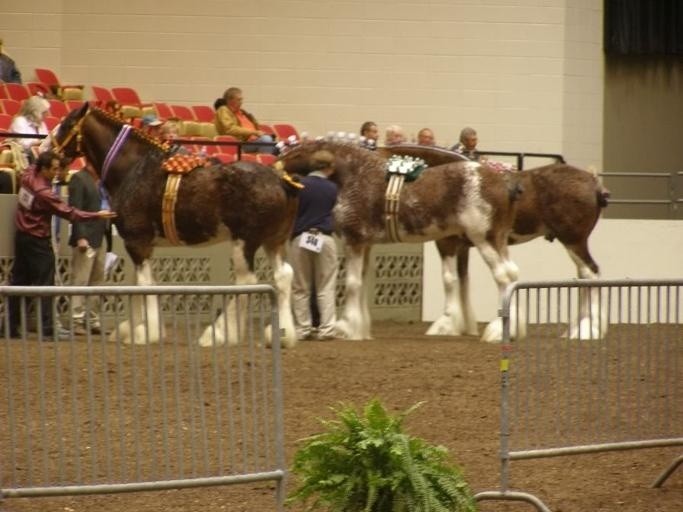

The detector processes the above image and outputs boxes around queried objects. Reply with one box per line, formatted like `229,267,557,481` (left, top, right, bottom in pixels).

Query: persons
7,95,51,152
0,53,22,83
0,152,119,340
286,149,339,340
449,127,480,162
215,86,275,155
384,125,404,146
418,128,434,147
67,156,114,335
139,115,168,145
360,121,379,142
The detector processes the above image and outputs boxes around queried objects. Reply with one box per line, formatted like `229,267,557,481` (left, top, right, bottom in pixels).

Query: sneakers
43,326,112,336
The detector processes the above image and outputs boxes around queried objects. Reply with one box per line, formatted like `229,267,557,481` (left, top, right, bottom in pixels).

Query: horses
37,101,302,351
371,142,611,340
267,138,522,344
